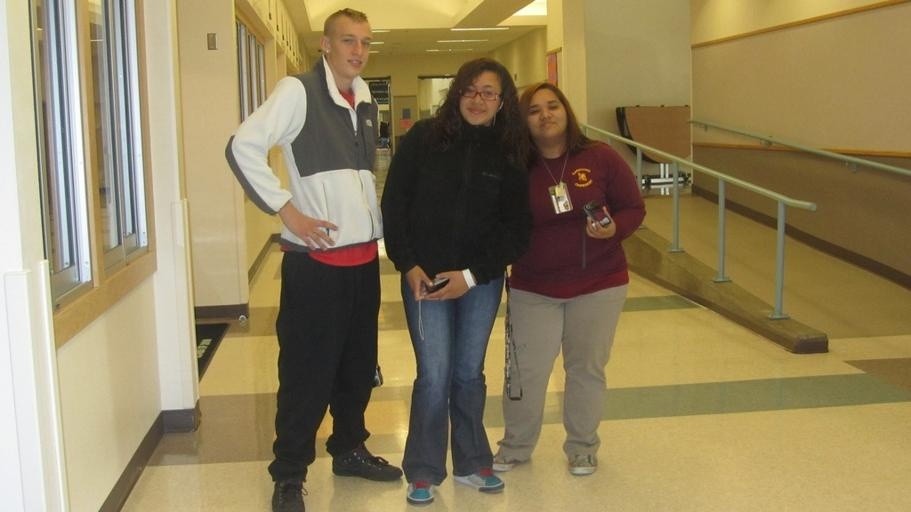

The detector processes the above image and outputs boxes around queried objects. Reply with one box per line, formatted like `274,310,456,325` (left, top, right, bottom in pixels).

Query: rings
311,232,315,238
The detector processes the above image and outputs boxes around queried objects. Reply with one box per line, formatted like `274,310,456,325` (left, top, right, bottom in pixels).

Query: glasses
458,86,503,100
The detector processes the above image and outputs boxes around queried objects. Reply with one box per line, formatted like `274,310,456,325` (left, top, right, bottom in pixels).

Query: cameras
427,278,448,293
582,200,611,227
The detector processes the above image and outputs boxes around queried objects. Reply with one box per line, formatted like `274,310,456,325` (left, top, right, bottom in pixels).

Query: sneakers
270,478,309,512
406,478,437,504
454,467,505,494
331,446,404,483
568,454,598,476
489,453,533,471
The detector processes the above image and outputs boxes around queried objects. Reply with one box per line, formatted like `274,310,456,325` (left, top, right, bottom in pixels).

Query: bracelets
462,269,475,289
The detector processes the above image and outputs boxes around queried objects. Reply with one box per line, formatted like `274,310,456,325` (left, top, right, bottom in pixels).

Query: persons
490,82,645,474
224,7,404,512
381,57,535,506
